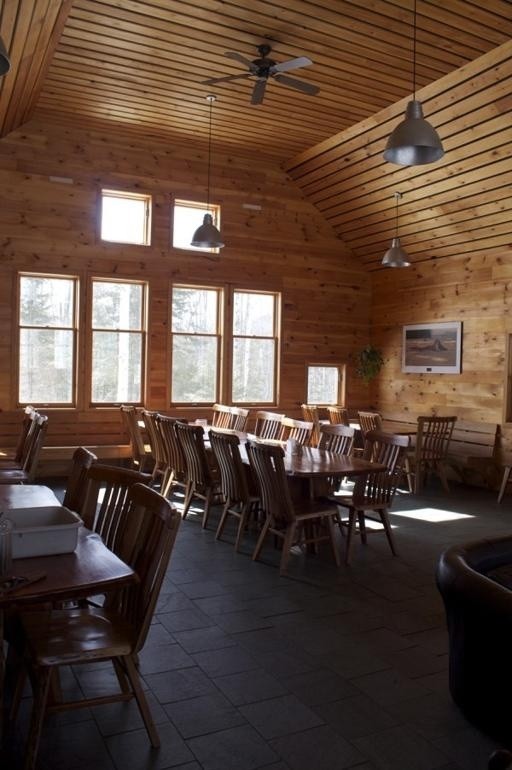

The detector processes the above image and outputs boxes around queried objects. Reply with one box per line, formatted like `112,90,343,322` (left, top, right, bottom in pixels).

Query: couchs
430,533,512,749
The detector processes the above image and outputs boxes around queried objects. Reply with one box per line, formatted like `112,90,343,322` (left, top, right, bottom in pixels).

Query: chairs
358,409,388,460
244,438,343,578
210,404,234,430
300,404,325,447
4,480,181,763
280,418,313,445
0,405,39,458
398,416,456,497
318,424,356,455
208,430,261,552
151,411,189,498
491,421,511,504
141,412,165,496
0,415,48,482
0,484,142,605
171,419,220,529
62,448,95,515
255,410,284,440
227,406,249,431
52,464,150,702
327,407,347,425
326,430,410,555
118,405,148,467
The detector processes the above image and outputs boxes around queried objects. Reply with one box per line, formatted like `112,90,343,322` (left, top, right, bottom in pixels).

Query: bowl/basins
0,506,83,558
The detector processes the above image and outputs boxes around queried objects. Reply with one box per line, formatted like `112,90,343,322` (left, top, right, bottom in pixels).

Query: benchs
379,408,499,500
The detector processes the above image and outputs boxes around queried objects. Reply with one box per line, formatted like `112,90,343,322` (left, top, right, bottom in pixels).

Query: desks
177,421,388,553
320,418,452,437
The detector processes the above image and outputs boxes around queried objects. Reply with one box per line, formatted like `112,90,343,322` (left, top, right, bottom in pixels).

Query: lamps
383,1,444,167
379,189,411,270
191,95,226,250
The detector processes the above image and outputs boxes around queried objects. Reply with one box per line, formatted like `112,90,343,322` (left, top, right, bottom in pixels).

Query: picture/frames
402,320,460,374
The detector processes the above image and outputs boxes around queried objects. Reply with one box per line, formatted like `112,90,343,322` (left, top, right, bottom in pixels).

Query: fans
203,43,322,107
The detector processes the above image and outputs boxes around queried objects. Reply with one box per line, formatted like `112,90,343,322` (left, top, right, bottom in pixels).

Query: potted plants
354,345,385,386
